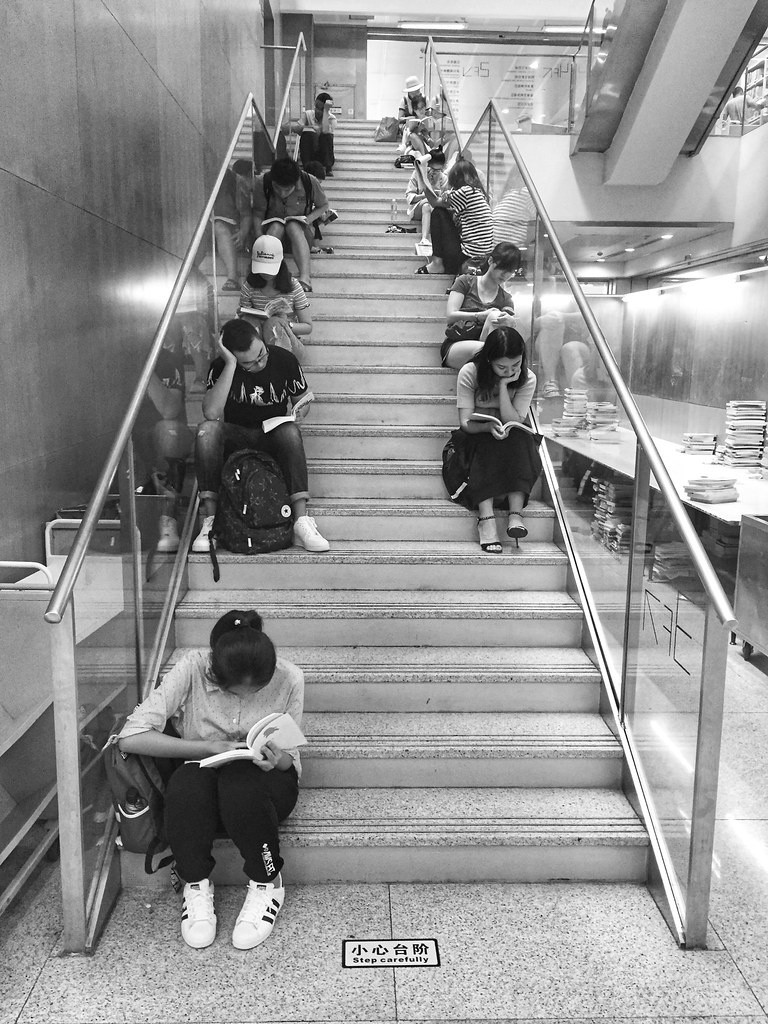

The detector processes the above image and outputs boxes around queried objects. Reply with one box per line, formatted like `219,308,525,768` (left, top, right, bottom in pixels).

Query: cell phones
501,309,517,315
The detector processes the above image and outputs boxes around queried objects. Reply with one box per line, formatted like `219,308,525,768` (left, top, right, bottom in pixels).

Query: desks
537,423,768,525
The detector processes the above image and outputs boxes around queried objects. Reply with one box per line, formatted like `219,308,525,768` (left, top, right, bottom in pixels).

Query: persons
456,326,544,553
192,168,250,291
190,318,330,553
233,234,314,366
115,609,305,951
102,348,188,554
398,76,431,136
439,241,522,370
166,268,219,391
252,157,329,292
291,93,337,180
405,144,449,246
492,164,536,247
400,95,436,157
415,159,494,274
534,279,599,399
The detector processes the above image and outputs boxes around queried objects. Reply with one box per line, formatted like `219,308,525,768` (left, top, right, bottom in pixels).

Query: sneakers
293,514,330,552
179,878,216,948
232,871,290,952
158,515,181,551
192,515,217,551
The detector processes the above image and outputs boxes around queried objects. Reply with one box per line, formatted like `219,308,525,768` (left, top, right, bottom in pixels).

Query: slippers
223,279,242,290
298,280,313,292
415,264,446,274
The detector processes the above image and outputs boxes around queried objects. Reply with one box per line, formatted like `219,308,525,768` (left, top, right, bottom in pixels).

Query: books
261,214,309,228
241,297,294,320
210,216,237,226
302,127,317,133
261,392,315,434
409,116,434,133
199,712,308,769
470,413,536,436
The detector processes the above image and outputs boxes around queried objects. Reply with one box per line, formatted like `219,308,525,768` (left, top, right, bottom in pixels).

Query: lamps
542,23,619,33
397,19,469,29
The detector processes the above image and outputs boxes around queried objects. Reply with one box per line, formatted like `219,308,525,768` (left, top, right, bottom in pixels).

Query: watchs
288,320,293,329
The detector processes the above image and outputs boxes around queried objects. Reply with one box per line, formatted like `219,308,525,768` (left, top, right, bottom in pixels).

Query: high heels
476,516,503,553
507,512,528,548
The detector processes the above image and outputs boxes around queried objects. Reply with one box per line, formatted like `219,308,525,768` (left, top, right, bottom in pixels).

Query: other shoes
418,239,432,246
543,379,561,398
396,144,406,152
325,170,333,177
315,247,334,255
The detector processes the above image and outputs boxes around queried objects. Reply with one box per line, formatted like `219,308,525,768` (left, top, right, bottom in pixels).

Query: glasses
243,347,270,373
428,166,443,173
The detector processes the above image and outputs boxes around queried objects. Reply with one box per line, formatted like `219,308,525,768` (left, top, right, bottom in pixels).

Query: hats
403,77,423,92
252,234,283,275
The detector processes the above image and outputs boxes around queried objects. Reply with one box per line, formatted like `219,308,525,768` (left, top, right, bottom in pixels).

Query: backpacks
105,713,175,873
209,448,295,582
441,427,475,512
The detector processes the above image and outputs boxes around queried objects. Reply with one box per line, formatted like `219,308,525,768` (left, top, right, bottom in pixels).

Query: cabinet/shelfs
0,520,129,914
746,59,768,138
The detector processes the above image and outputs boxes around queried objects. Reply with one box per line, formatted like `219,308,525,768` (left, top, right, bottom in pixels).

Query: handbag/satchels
394,154,416,169
374,117,399,142
